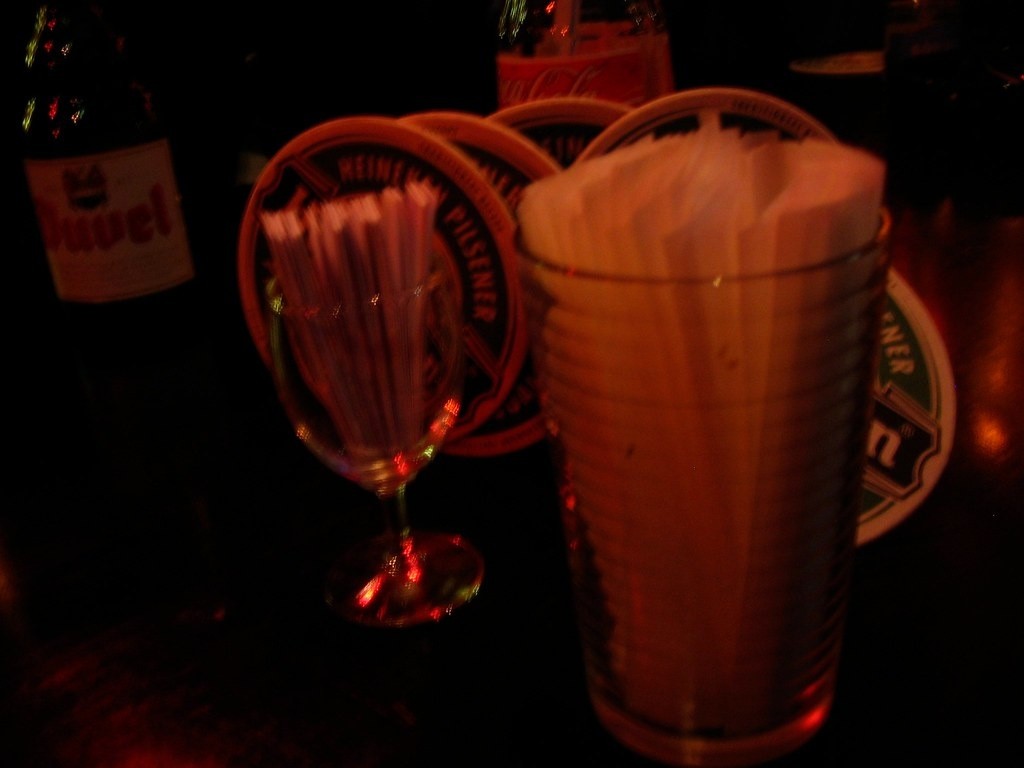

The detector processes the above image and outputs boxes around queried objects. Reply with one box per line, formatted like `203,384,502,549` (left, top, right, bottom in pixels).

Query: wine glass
253,178,485,630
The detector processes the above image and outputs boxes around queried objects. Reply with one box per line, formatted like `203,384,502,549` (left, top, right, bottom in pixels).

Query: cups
510,206,897,767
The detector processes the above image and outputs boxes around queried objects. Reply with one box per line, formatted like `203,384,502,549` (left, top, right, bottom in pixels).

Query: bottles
18,0,202,318
889,198,1024,494
494,1,676,116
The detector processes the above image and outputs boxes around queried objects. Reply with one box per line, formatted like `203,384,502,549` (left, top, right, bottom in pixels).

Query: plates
235,91,957,555
